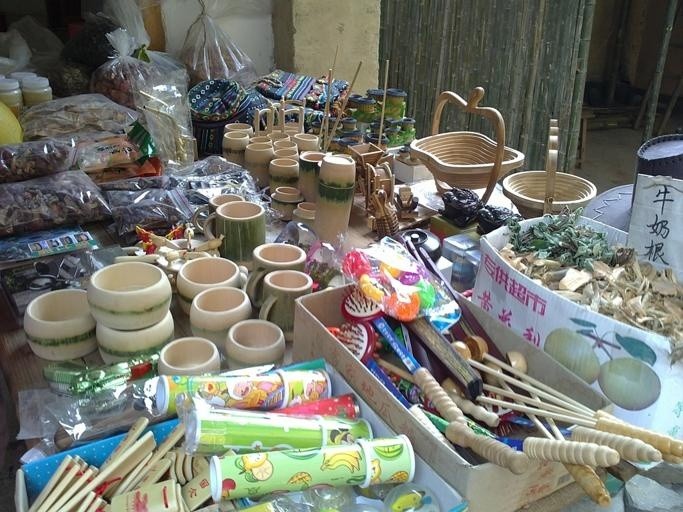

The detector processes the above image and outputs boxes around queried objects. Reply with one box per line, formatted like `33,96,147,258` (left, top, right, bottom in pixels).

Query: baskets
409,87,524,209
503,119,597,219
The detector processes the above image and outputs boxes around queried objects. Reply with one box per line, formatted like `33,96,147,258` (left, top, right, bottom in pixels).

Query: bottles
0,79,23,116
21,76,52,107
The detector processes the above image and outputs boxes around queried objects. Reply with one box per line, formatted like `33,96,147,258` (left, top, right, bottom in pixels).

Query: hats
581,133,683,234
186,80,277,156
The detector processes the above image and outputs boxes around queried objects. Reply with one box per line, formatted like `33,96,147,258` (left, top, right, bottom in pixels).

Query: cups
195,194,245,235
204,201,265,272
259,271,311,342
245,243,309,311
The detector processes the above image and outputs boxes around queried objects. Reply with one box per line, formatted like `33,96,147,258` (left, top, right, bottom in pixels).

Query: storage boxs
470,212,683,442
292,282,613,512
14,358,469,512
